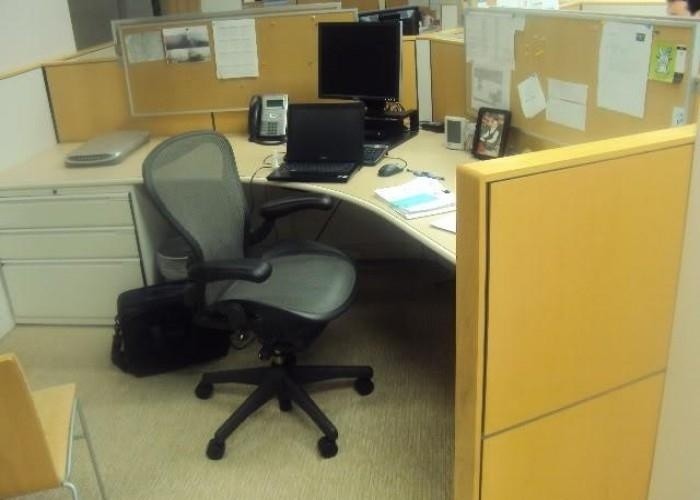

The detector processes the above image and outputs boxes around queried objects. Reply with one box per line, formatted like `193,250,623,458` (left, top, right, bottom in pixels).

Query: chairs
1,352,106,500
141,129,375,461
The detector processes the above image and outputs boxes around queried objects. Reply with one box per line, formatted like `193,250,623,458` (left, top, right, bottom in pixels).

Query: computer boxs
363,109,419,149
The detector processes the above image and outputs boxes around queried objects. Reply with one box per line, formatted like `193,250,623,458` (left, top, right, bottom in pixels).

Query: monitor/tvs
358,6,419,35
319,21,403,112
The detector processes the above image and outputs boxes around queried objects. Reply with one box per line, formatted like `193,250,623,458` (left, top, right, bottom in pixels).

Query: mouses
379,164,400,176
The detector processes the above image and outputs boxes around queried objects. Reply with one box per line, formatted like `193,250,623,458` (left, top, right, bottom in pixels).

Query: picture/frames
471,107,513,161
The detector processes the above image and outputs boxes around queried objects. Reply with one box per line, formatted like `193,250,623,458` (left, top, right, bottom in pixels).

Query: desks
0,125,493,328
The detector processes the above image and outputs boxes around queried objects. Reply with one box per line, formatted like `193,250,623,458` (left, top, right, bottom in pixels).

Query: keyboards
363,144,389,164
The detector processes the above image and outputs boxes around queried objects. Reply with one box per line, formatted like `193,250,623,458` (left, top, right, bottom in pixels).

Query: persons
664,0,700,19
479,118,500,150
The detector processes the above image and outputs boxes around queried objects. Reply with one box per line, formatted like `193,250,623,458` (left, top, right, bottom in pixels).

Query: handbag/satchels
112,277,233,379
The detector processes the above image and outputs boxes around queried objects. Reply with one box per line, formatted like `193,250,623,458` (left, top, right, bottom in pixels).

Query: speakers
444,116,470,150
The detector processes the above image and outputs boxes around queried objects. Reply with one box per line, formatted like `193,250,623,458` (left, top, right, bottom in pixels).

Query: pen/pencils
407,169,444,180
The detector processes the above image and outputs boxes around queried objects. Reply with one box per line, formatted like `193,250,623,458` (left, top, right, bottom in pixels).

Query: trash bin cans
156,245,191,283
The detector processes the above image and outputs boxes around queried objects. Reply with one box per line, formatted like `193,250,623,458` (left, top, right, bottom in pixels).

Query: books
373,176,456,234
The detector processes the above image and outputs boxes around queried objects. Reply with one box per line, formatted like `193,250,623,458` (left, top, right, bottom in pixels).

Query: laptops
266,103,363,183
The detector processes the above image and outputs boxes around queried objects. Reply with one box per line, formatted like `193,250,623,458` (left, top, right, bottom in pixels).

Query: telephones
249,94,289,142
